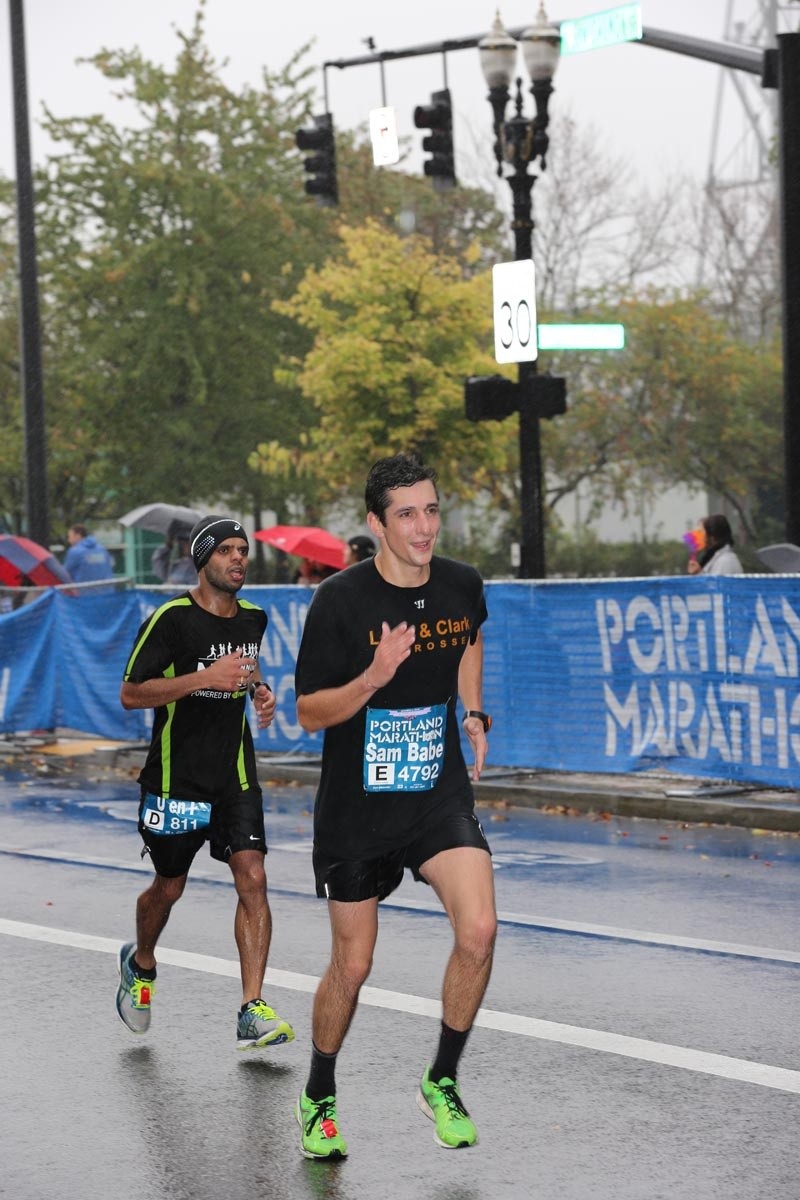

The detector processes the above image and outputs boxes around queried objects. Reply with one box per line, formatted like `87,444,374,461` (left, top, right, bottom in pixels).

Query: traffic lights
414,89,459,187
294,113,339,205
464,371,567,423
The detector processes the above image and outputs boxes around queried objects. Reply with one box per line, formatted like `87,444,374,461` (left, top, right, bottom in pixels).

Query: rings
240,677,243,684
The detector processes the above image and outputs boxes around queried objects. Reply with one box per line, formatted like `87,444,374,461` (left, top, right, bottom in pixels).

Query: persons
292,536,377,587
686,515,745,577
295,455,497,1165
149,535,200,595
62,522,115,596
10,574,41,612
115,516,294,1052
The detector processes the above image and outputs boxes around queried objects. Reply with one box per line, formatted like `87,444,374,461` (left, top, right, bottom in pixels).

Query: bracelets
364,669,379,689
248,680,272,700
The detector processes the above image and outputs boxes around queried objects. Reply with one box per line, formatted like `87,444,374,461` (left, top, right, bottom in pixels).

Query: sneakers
114,943,157,1034
294,1082,348,1160
236,999,295,1050
414,1064,477,1148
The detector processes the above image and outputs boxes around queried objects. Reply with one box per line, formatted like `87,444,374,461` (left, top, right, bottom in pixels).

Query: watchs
462,710,492,732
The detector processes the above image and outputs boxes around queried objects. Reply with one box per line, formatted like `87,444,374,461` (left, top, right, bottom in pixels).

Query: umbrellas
252,524,349,570
0,535,81,596
118,503,205,537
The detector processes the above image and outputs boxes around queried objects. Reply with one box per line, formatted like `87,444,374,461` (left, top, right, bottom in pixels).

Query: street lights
477,0,562,582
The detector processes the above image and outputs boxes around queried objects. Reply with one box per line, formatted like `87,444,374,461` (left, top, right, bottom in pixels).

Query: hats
189,516,249,573
